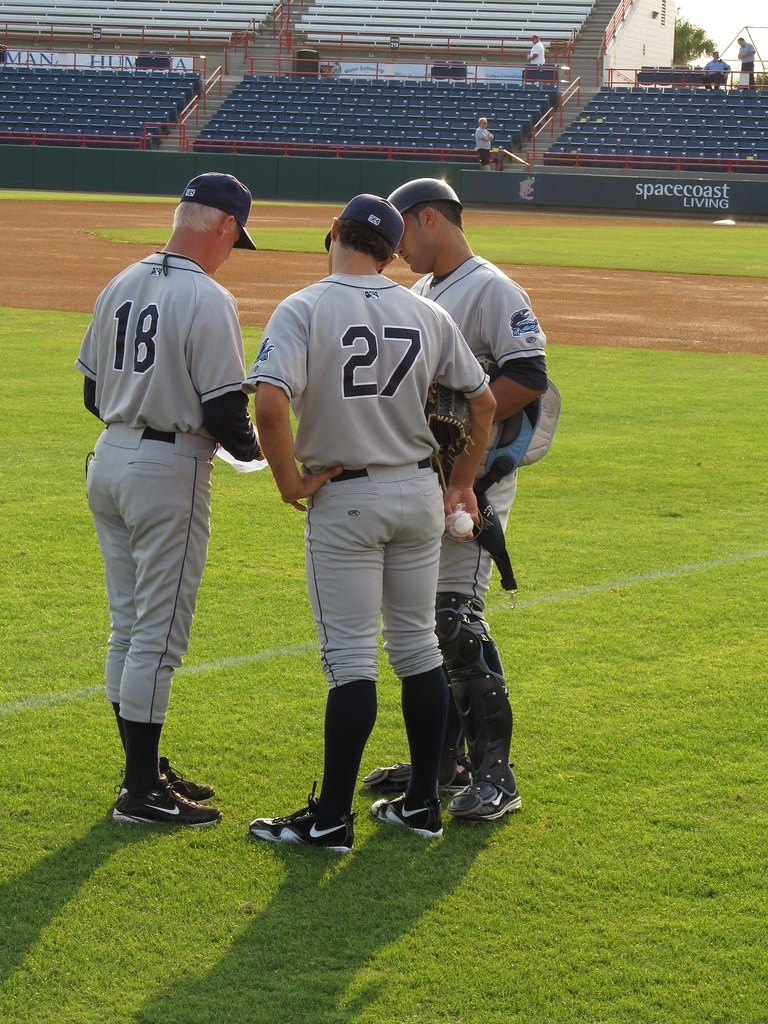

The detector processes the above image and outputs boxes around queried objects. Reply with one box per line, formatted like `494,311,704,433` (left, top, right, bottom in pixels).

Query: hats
180,171,257,250
325,194,405,273
531,34,539,40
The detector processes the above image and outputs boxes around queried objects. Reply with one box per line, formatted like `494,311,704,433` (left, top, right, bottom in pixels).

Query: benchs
0,0,284,46
289,0,601,52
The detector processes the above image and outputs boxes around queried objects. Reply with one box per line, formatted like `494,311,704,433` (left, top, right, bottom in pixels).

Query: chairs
0,65,768,177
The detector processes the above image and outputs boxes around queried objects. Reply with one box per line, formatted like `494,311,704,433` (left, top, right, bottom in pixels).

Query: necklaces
155,251,205,277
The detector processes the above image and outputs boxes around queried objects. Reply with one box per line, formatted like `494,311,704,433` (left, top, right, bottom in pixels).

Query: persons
703,51,731,90
240,193,497,852
475,117,494,171
527,34,545,64
363,177,548,820
737,38,755,90
74,172,265,828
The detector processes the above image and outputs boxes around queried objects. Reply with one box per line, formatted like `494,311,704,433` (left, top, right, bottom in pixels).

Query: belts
330,457,431,482
106,422,176,444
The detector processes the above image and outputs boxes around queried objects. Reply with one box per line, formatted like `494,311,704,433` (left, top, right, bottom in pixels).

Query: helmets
386,178,463,214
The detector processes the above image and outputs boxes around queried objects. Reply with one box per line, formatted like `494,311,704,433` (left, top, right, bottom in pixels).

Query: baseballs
449,510,474,538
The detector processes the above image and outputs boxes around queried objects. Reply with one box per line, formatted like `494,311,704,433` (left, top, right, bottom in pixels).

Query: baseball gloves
423,382,471,458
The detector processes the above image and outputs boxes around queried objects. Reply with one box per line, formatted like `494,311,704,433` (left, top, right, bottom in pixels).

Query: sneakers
458,768,522,819
159,756,215,805
249,781,358,854
113,773,223,825
370,793,444,838
372,753,471,791
